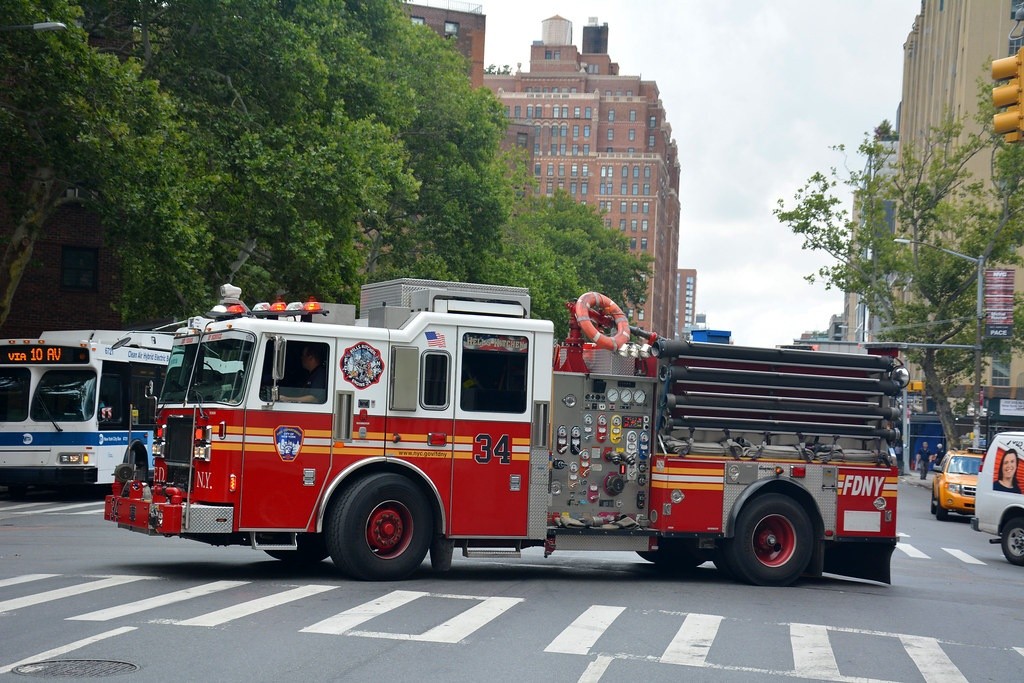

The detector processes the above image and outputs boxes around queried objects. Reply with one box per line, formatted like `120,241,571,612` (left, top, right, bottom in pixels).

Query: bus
0,329,244,488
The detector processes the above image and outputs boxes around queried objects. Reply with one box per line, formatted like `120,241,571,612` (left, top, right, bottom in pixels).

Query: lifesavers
575,291,631,350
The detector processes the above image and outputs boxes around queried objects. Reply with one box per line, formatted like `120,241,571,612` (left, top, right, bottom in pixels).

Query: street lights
894,238,983,454
839,325,910,476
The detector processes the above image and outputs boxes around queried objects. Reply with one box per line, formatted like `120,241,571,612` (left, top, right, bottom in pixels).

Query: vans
970,431,1024,567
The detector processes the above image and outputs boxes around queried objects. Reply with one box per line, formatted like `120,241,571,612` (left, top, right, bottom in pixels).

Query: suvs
931,447,985,520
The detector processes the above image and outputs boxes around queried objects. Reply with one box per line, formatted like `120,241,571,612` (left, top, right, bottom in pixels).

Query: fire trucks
103,277,909,588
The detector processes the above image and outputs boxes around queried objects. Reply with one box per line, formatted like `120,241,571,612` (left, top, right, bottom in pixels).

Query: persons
915,441,933,480
993,448,1022,494
894,442,903,466
935,443,945,466
277,342,329,404
